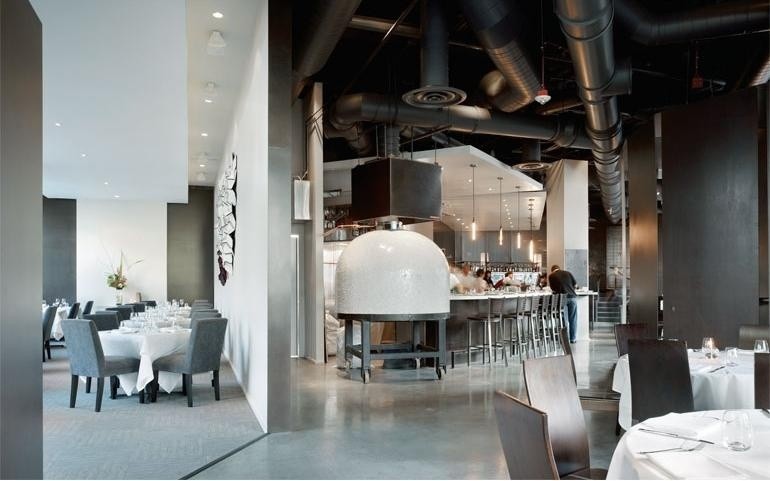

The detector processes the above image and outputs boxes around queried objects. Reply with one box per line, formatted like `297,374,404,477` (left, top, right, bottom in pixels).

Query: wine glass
42,298,69,309
120,299,188,335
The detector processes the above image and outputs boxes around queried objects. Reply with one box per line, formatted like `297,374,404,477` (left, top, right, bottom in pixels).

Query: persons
549,265,578,343
450,263,522,295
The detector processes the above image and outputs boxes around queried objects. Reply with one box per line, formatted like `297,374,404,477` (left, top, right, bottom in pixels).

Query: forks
637,442,707,455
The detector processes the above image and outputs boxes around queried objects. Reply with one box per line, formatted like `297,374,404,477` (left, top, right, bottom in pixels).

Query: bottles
461,262,538,272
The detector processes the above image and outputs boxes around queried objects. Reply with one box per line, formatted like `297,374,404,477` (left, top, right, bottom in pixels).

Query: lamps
534,1,552,105
468,163,533,262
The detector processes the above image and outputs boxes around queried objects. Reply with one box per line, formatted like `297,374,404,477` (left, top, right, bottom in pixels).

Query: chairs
523,354,607,480
493,389,585,480
467,293,568,367
754,352,770,409
559,327,622,436
614,322,649,436
627,337,695,426
43,300,228,411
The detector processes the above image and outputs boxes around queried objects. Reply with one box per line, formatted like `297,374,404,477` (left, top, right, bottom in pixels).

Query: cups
722,409,752,452
754,340,768,353
725,346,738,367
582,287,588,292
466,283,535,295
702,337,715,355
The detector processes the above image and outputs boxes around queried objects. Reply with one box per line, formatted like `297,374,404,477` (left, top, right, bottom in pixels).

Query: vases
115,289,123,305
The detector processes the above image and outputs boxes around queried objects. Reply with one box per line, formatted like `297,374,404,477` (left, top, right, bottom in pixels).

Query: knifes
709,366,726,373
638,428,715,444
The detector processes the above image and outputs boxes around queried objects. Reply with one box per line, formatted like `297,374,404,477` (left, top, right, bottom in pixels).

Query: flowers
105,248,144,289
